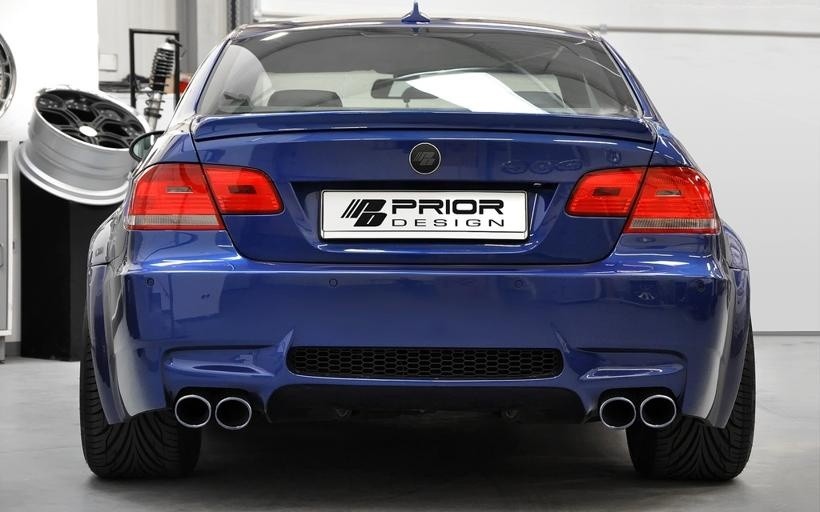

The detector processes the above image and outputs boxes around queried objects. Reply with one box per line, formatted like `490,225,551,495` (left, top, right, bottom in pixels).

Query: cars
78,1,756,485
